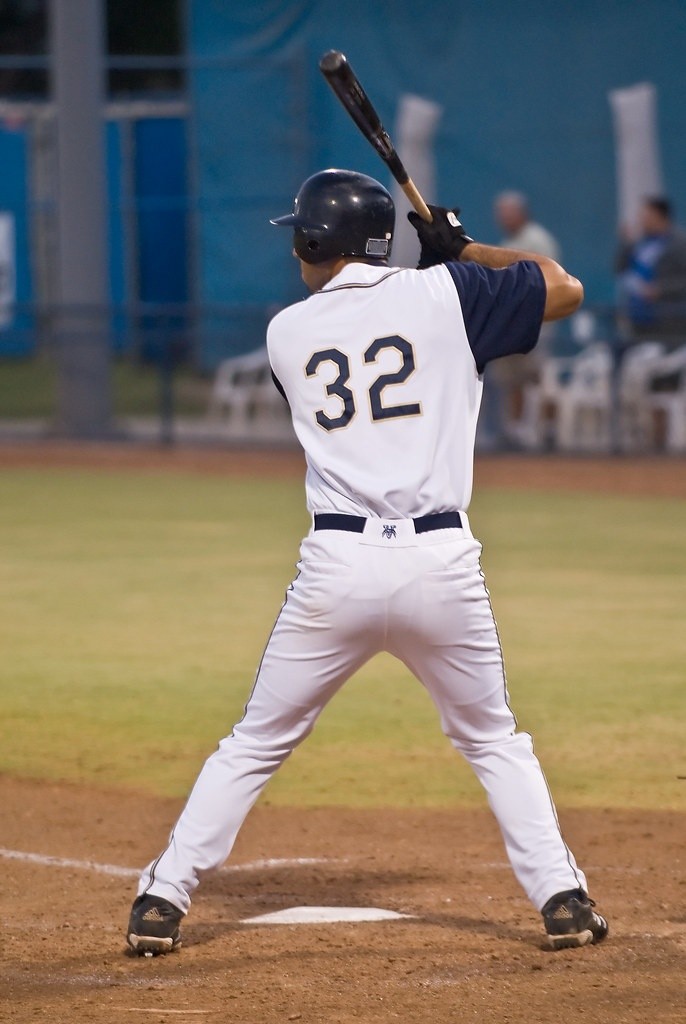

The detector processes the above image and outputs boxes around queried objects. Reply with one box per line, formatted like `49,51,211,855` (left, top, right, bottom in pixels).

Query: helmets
269,169,395,265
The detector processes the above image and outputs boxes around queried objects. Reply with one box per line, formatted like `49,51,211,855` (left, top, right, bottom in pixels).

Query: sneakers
544,889,609,949
127,894,185,952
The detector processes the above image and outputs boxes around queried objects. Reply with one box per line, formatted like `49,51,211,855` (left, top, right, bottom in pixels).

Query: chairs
208,342,686,453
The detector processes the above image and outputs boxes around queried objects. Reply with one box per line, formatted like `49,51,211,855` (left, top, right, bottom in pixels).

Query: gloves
406,205,474,269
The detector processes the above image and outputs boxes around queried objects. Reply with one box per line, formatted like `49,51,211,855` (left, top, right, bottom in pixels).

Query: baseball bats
319,50,433,225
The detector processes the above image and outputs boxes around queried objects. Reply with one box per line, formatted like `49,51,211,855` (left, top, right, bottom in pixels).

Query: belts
314,512,462,533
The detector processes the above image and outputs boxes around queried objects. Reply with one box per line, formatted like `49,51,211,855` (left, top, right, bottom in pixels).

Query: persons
608,193,684,454
126,167,607,952
493,186,561,421
